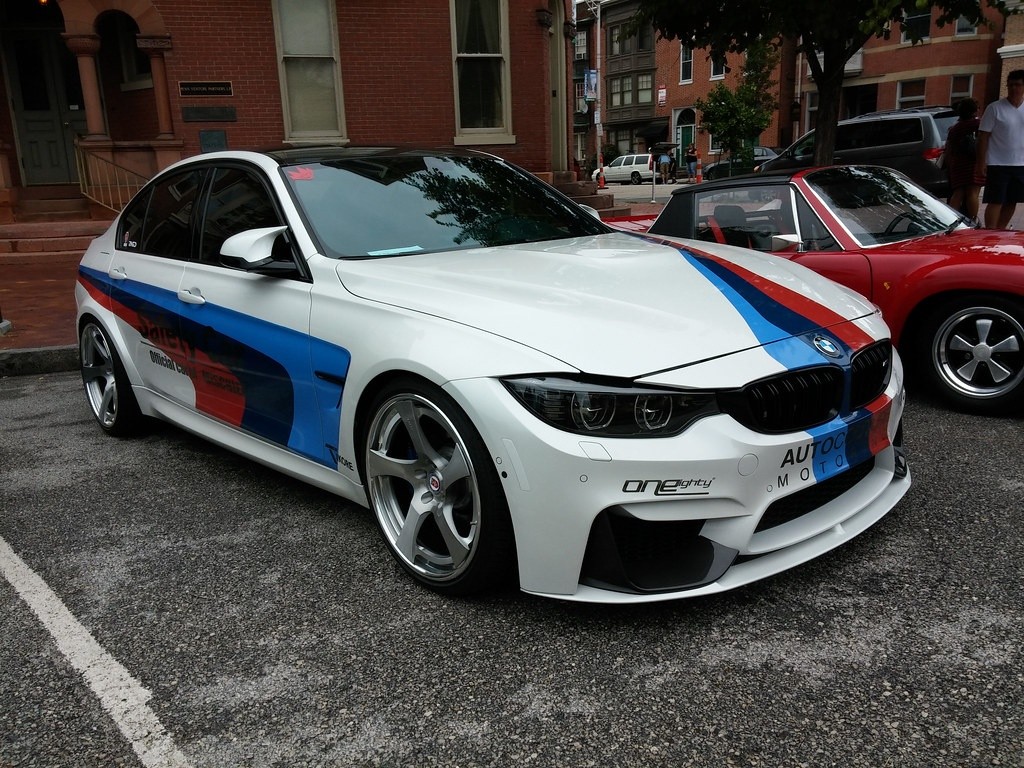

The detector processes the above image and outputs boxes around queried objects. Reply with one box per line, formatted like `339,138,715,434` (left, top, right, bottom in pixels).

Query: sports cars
596,164,1023,420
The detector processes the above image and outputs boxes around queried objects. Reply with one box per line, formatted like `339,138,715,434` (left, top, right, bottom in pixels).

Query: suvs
590,141,680,187
751,105,963,199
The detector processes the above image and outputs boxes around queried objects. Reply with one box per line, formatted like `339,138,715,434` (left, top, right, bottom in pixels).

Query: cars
74,145,912,608
704,145,785,181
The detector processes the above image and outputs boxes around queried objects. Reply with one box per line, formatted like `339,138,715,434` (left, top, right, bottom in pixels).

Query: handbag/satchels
966,123,979,165
936,153,948,171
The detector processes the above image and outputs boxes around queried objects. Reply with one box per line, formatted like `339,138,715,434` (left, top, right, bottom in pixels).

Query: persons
684,143,697,183
946,98,987,217
657,151,678,184
976,70,1024,230
